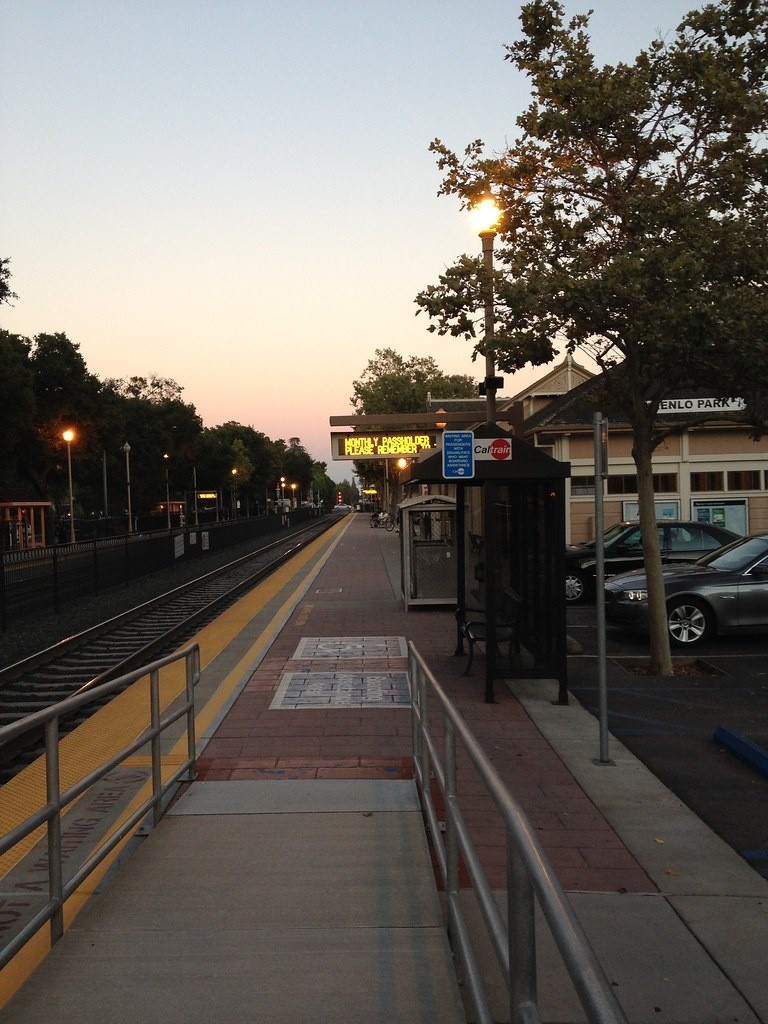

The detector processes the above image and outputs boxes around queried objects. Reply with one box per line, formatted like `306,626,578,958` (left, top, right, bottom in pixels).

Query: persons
423,514,432,539
371,506,388,528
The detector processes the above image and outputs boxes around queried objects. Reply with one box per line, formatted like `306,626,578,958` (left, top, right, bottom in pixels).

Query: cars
605,532,768,649
565,518,745,606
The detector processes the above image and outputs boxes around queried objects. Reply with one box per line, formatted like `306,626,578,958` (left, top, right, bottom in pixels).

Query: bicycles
368,508,396,531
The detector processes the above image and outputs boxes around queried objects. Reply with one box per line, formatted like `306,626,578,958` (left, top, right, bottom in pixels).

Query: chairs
455,587,524,676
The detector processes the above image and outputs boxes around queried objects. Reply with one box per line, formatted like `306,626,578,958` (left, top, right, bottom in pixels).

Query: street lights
280,476,297,513
123,441,133,534
62,429,75,543
163,453,172,528
468,193,503,436
231,468,238,519
